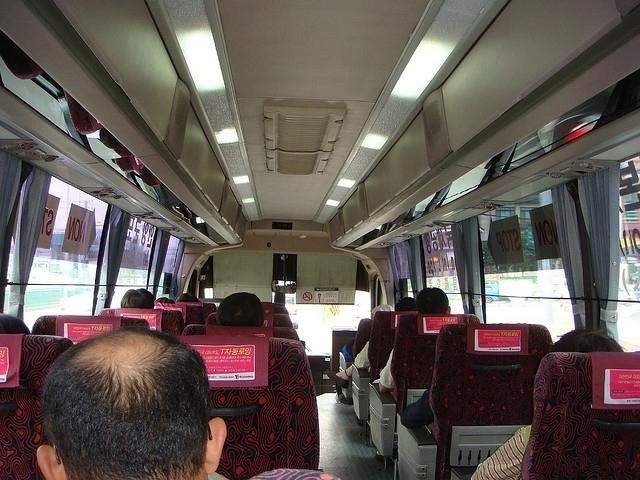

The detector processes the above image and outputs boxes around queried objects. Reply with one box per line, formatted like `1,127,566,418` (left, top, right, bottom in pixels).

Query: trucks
482,278,536,303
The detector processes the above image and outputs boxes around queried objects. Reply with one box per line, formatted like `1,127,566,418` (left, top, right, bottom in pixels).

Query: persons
34,326,230,480
1,288,451,429
469,327,623,480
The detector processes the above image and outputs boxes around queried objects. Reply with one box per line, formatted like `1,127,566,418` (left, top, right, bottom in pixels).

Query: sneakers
327,372,349,388
